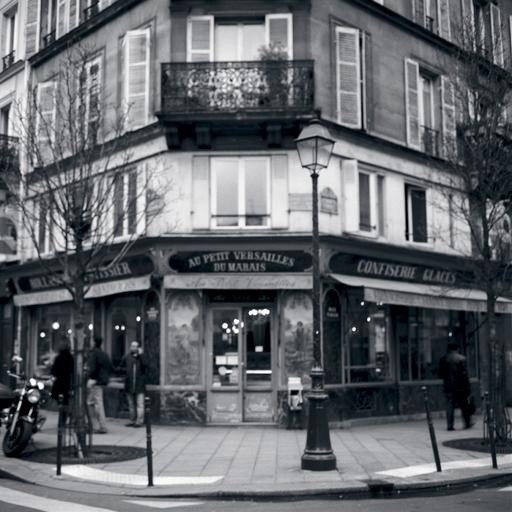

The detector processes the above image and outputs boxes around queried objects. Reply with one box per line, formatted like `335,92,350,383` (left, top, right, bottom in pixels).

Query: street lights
294,112,338,472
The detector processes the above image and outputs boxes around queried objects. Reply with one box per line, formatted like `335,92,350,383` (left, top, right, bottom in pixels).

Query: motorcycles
3,370,51,457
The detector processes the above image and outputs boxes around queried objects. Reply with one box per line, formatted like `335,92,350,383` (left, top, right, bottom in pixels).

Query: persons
437,341,476,431
116,341,146,428
81,334,112,434
294,321,308,348
49,342,76,418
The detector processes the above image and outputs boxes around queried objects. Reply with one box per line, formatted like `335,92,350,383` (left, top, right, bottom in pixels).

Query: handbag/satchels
466,393,476,416
